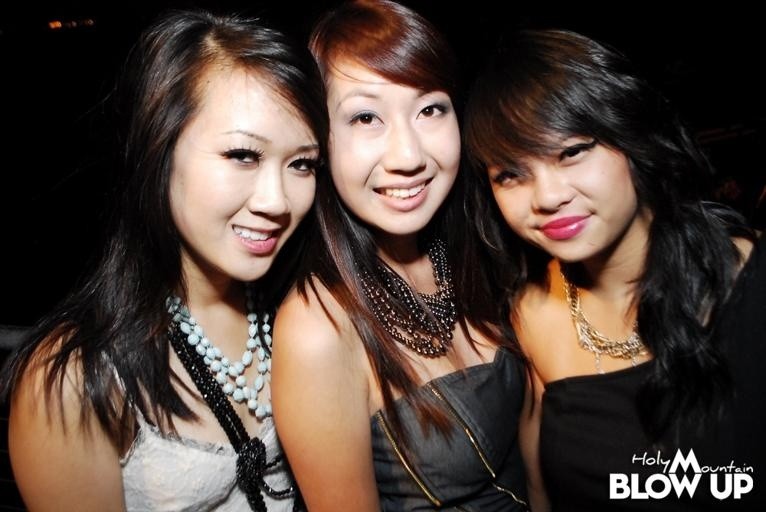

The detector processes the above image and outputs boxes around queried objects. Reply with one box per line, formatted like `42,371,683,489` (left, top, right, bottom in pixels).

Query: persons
443,26,766,512
268,0,553,509
5,2,309,512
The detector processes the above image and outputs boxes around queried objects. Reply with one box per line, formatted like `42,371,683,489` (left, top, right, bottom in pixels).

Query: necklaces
350,231,456,358
563,277,648,371
166,290,276,426
